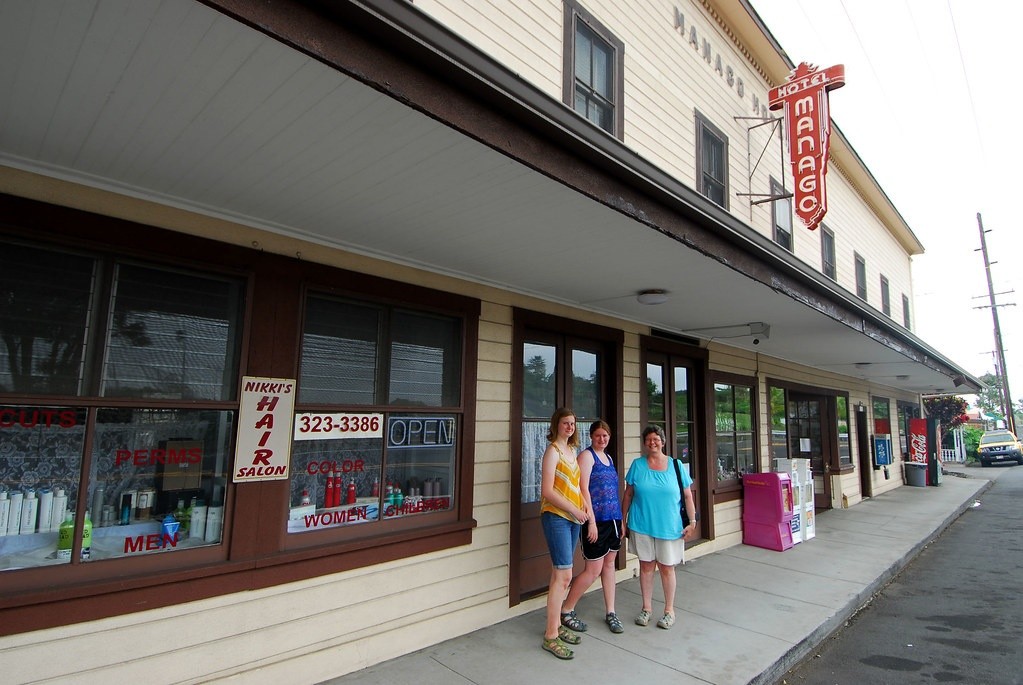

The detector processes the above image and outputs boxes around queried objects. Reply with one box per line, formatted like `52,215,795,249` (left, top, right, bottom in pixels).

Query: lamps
579,289,672,306
876,374,910,382
814,363,873,372
681,322,771,345
949,373,967,387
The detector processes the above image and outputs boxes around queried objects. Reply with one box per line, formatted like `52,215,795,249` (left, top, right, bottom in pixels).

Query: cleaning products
189,499,223,547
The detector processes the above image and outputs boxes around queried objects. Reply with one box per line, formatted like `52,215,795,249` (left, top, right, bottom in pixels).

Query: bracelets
689,519,696,524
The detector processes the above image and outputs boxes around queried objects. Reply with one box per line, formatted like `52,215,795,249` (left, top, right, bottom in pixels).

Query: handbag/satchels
680,505,700,529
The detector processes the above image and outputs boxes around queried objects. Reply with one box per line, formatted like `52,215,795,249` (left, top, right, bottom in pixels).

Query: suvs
975,428,1023,469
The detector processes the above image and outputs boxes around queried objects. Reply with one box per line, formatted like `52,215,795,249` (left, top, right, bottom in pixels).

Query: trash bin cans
903,461,929,488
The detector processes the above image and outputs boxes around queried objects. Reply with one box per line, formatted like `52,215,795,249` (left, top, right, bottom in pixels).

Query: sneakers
561,610,588,631
657,611,677,628
635,608,653,625
606,612,625,633
542,636,574,660
557,625,581,644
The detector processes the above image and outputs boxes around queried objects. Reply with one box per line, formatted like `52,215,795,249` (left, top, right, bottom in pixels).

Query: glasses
645,438,662,443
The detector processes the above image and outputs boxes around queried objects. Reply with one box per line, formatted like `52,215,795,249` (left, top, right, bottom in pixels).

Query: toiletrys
372,477,379,497
334,472,342,508
0,484,73,537
301,491,311,507
423,477,443,511
385,481,403,510
324,470,334,508
91,484,157,528
158,498,197,550
56,508,93,560
347,476,356,505
405,487,423,513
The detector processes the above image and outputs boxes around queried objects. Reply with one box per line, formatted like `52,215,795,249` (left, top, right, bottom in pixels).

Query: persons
538,406,591,660
559,420,625,634
621,424,697,631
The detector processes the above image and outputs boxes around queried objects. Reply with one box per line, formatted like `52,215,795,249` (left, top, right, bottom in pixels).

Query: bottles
325,470,341,508
347,477,356,504
423,477,440,497
57,509,93,561
372,477,380,498
174,496,223,544
301,490,310,506
386,481,423,514
0,489,67,535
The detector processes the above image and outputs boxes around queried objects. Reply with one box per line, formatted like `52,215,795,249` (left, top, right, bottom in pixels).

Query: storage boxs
773,458,816,544
